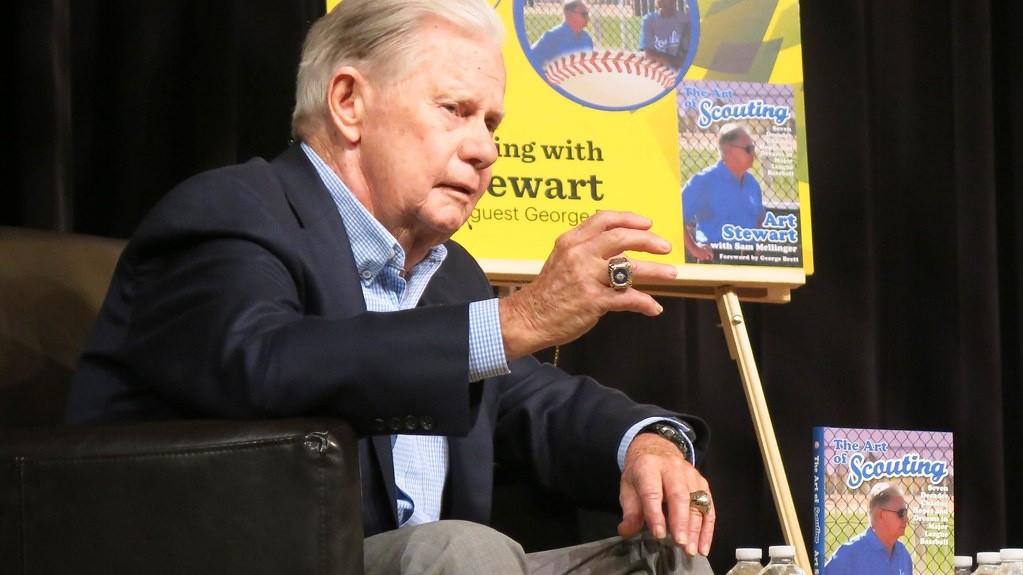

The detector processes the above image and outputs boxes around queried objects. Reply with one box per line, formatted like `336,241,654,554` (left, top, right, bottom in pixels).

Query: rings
690,490,711,513
608,257,632,289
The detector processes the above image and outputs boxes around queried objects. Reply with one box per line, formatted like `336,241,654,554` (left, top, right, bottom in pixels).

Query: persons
682,123,767,265
63,0,715,575
824,482,912,575
529,0,593,66
639,0,690,67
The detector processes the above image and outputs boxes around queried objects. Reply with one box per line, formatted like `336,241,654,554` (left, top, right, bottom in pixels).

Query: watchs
637,423,692,462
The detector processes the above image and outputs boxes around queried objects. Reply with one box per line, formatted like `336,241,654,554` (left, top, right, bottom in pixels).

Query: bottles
969,548,1023,575
953,555,973,575
726,548,765,575
759,545,806,575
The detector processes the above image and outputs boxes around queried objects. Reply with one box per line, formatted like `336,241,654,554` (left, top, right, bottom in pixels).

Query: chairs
0,227,583,575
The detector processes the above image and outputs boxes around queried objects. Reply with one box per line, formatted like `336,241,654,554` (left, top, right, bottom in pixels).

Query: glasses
881,508,908,518
724,140,757,154
570,10,590,18
